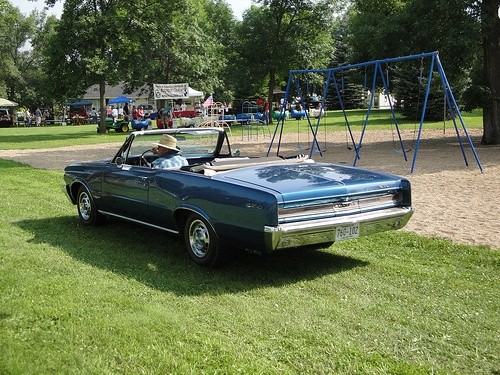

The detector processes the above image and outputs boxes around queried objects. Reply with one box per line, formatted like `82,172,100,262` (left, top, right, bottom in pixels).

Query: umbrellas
108,96,135,104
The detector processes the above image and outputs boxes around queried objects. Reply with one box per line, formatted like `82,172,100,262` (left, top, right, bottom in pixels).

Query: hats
152,134,183,152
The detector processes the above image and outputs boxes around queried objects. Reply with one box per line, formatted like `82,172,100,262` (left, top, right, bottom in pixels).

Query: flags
203,95,213,107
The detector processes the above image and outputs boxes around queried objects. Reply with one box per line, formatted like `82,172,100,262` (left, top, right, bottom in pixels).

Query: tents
189,87,203,112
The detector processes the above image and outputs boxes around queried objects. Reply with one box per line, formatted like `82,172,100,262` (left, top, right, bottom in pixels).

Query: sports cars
62,125,414,268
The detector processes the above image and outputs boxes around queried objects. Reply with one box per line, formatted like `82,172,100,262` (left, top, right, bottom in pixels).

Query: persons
152,134,189,170
159,101,187,128
24,108,50,127
274,102,322,120
68,107,96,124
97,102,144,124
257,95,269,124
189,114,203,119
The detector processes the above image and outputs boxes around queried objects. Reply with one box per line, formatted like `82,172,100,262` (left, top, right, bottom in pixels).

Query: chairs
137,117,141,122
116,115,125,122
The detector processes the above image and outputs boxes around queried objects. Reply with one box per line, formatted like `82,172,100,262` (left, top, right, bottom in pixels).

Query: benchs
13,120,63,127
